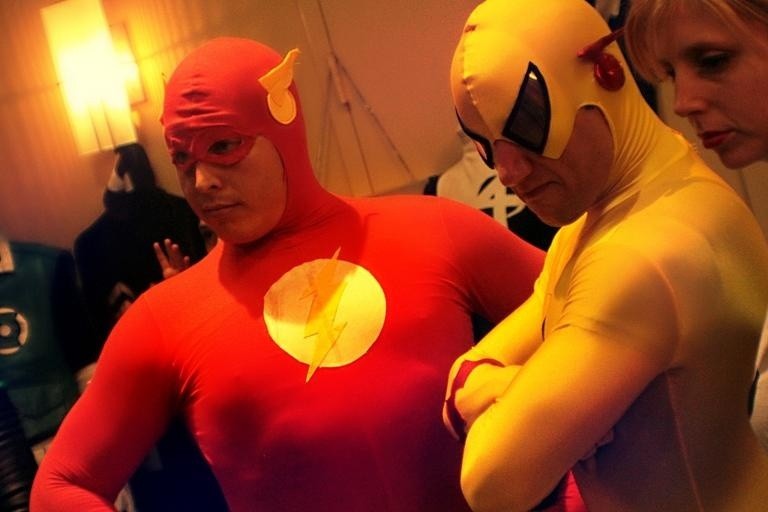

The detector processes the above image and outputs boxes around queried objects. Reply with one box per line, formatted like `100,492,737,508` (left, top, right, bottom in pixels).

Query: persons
151,205,219,281
29,34,557,511
443,0,768,512
625,0,768,447
424,124,557,250
